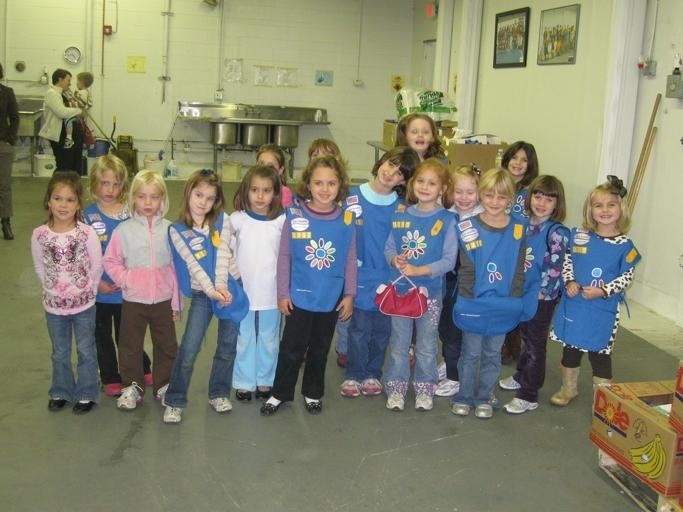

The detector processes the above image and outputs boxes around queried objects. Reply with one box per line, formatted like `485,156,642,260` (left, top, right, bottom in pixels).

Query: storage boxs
668,360,683,432
33,154,56,176
448,142,507,171
590,379,683,497
382,120,396,146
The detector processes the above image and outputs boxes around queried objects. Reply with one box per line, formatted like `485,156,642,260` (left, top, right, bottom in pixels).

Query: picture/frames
492,7,531,69
537,3,581,66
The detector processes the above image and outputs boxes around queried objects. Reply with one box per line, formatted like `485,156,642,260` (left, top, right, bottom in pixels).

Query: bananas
629,432,668,481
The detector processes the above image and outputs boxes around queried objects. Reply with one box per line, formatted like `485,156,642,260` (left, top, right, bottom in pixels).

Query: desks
367,141,391,162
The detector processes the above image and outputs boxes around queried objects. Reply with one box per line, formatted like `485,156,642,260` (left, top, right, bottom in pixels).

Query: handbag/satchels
372,276,428,319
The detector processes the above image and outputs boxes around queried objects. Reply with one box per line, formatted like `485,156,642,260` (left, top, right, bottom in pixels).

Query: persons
498,175,569,415
543,26,576,60
162,144,293,422
450,167,528,418
498,22,523,49
102,169,184,409
64,71,94,149
80,155,153,397
499,141,538,364
550,175,641,406
0,62,20,240
38,69,87,209
262,112,485,415
30,171,104,413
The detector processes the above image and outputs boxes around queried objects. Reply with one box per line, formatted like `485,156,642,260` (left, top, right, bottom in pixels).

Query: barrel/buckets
87,140,110,157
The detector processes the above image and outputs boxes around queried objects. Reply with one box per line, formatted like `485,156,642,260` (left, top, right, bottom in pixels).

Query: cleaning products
166,160,176,179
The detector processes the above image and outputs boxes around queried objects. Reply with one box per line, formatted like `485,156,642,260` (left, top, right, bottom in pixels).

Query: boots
591,376,610,391
550,362,580,405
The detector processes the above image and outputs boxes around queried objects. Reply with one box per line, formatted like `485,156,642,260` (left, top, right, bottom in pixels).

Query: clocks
64,47,81,65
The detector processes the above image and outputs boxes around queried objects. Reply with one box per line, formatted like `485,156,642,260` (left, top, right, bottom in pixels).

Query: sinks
240,119,268,147
209,117,239,145
270,120,299,148
14,97,44,137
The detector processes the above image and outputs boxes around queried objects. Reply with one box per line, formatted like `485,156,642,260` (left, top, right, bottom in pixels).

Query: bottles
41,69,48,85
166,160,178,179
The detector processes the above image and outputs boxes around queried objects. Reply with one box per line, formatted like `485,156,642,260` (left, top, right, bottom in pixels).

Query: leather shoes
255,386,271,399
260,395,281,415
47,396,67,412
303,396,321,414
236,389,251,402
73,400,95,412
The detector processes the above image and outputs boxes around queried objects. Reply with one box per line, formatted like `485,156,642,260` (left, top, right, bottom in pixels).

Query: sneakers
103,383,122,396
360,378,381,396
414,393,433,411
473,404,492,419
208,395,231,412
163,403,181,424
143,374,153,386
436,361,447,380
385,393,405,411
2,217,14,238
116,382,142,410
153,383,169,398
337,352,348,368
497,376,520,390
63,138,73,149
504,398,537,414
451,402,469,415
340,380,360,397
434,380,458,397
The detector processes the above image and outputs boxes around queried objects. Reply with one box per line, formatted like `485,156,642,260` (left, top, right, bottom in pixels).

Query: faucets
235,102,261,118
